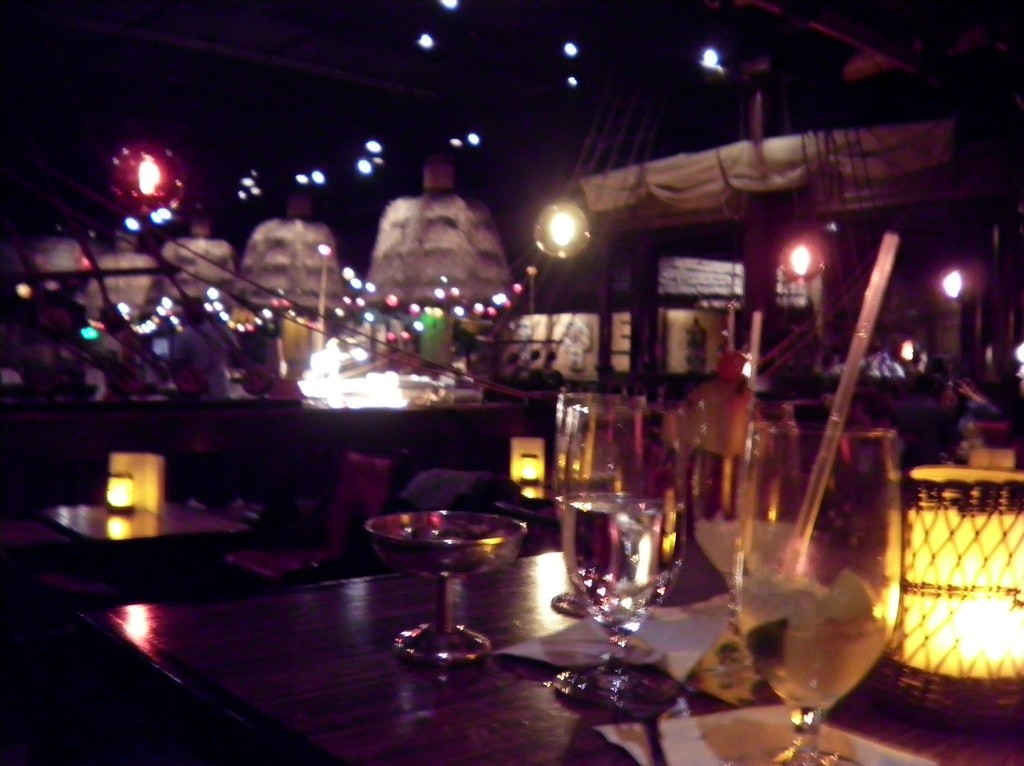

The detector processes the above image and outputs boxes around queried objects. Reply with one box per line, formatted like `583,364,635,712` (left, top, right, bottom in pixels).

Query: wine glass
737,422,901,766
365,513,526,666
550,494,688,716
694,403,794,697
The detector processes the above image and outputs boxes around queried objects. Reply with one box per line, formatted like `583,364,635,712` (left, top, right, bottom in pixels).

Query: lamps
872,465,1024,732
521,453,540,486
104,471,136,516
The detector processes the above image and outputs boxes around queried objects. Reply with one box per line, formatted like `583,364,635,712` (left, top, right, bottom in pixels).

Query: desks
40,502,249,545
74,561,1024,766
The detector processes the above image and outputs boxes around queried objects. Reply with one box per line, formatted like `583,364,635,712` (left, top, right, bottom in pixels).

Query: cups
556,392,689,604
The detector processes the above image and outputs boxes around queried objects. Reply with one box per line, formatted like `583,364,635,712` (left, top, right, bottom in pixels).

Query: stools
223,553,325,596
